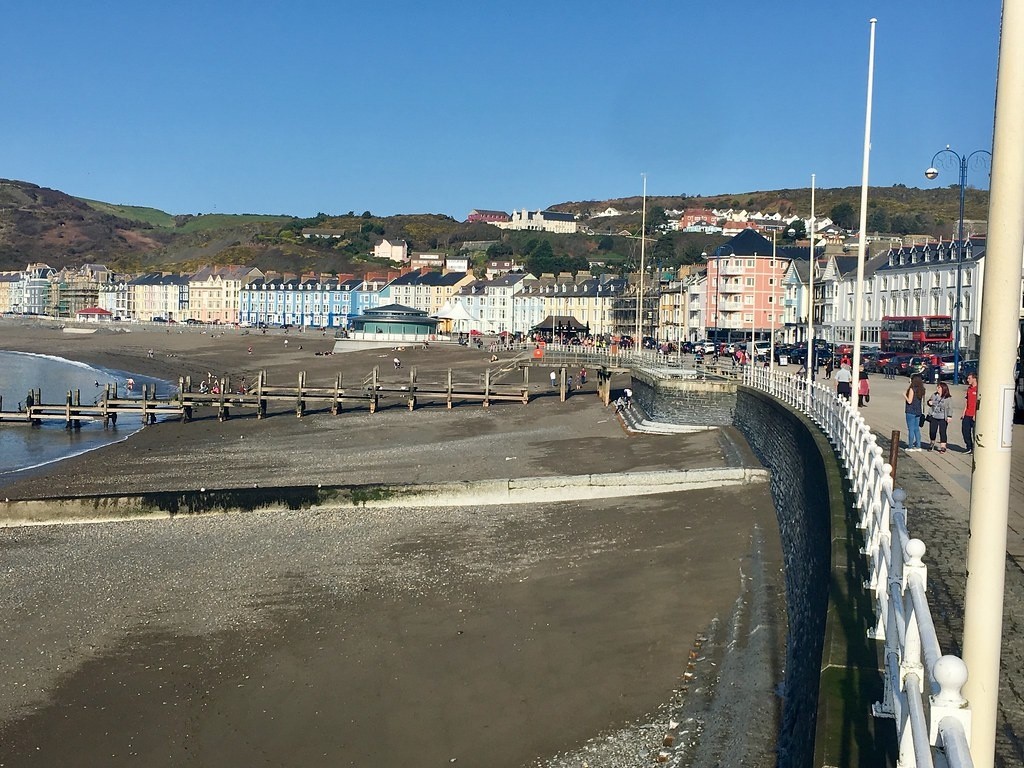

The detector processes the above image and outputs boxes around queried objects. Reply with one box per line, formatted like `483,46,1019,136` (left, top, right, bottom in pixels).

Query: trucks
747,340,771,359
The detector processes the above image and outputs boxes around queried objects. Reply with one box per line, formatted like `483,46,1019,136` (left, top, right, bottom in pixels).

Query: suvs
940,355,963,376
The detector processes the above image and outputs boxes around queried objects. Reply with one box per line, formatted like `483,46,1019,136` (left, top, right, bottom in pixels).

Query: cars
280,324,293,329
659,340,747,356
254,323,268,329
962,359,978,385
618,335,633,348
754,341,807,364
470,329,495,336
642,337,656,349
860,352,922,375
812,340,854,367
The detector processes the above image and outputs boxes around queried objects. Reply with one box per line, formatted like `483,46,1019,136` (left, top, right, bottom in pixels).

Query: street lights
924,144,992,385
505,278,522,334
700,244,735,358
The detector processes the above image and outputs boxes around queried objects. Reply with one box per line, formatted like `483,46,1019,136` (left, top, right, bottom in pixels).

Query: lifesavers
533,349,543,359
431,335,436,341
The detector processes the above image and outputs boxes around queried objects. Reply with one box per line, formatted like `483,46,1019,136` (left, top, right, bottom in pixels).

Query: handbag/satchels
824,365,828,369
919,414,926,428
926,415,934,423
865,389,870,402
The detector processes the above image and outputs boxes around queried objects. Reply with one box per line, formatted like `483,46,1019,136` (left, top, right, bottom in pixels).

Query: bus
881,315,954,354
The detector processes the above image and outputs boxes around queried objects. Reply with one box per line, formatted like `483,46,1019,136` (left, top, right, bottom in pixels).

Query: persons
903,374,925,451
927,382,953,453
497,331,553,349
836,355,871,408
695,348,704,367
198,371,220,394
238,378,249,394
393,357,400,366
458,332,483,348
550,367,588,393
314,351,332,356
823,357,833,379
422,340,427,349
284,339,289,347
392,346,405,352
614,388,632,414
298,345,303,350
735,349,757,366
553,333,634,349
644,341,691,355
960,372,977,455
248,346,253,355
147,349,153,358
126,377,134,389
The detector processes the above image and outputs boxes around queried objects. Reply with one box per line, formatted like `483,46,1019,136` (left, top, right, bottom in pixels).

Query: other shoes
905,448,922,452
962,448,973,456
929,443,934,451
940,447,946,454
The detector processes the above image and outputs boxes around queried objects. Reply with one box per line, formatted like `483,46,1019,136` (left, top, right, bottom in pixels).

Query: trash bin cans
930,364,941,384
